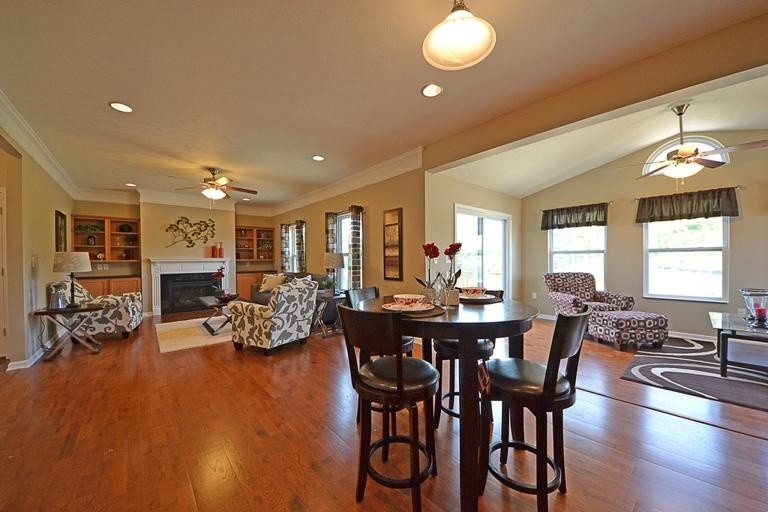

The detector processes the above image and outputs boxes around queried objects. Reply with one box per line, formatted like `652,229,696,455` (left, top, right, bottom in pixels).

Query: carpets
153,315,232,354
620,338,768,412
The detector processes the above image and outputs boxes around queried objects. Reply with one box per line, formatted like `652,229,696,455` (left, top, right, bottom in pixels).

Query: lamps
53,251,92,309
200,189,226,212
423,0,497,72
321,252,345,296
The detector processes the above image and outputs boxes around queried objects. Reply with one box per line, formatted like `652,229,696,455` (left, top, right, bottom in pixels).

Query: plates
458,293,495,300
381,302,434,312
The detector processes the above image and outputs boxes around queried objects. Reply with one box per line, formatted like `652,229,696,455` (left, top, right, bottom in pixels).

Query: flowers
412,242,464,287
211,266,226,296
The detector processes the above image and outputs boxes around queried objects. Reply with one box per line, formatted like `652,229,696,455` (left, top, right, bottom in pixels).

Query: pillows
259,273,312,294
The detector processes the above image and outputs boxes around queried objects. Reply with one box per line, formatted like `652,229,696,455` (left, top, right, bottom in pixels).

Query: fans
173,167,257,200
634,106,768,180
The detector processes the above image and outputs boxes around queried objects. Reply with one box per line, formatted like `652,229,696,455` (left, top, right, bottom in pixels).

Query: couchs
251,271,344,325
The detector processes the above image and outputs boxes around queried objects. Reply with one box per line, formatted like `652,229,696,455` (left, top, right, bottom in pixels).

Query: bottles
218,241,224,258
211,246,217,258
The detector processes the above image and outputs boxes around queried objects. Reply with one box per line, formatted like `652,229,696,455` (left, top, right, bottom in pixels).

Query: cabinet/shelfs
236,225,278,301
71,213,141,299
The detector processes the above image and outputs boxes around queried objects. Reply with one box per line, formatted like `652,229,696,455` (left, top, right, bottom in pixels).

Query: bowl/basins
461,288,487,297
214,293,239,303
96,252,104,260
392,294,425,309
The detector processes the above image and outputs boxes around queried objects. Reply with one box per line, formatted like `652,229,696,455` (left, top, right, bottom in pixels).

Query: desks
357,293,539,511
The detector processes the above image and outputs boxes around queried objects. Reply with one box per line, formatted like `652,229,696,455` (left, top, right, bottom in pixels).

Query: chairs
336,304,440,511
344,287,414,436
477,303,591,511
227,281,319,356
434,291,505,429
542,272,634,316
46,280,143,345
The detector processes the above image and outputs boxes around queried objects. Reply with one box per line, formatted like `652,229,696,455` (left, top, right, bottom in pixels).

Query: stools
590,309,670,351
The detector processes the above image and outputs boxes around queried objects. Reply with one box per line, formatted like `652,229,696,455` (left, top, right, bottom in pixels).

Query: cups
737,288,767,329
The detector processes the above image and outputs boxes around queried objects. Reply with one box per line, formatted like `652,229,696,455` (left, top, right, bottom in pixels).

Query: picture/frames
55,209,67,252
383,207,403,282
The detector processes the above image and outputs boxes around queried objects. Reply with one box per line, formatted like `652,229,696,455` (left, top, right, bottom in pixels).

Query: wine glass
439,271,455,310
